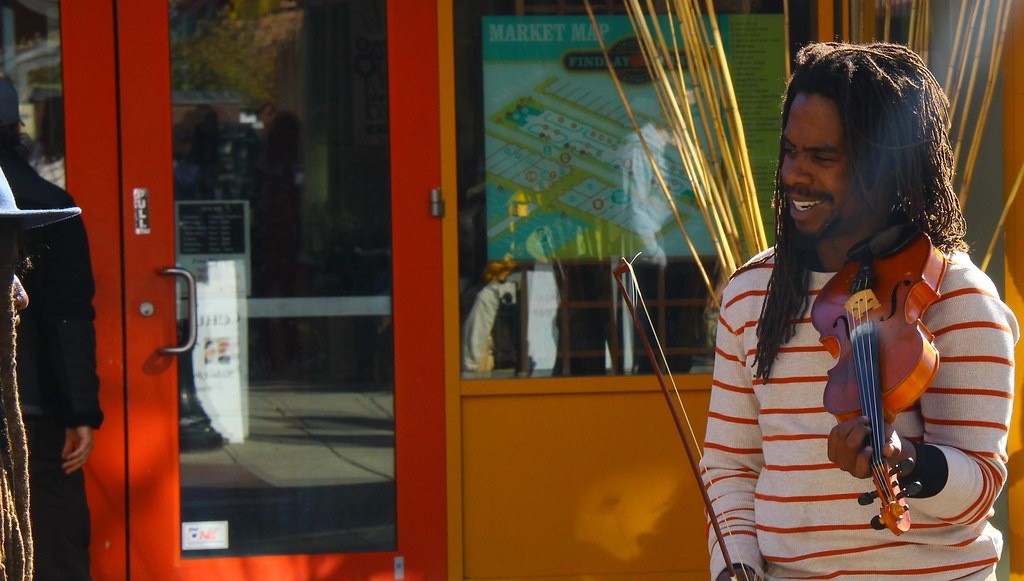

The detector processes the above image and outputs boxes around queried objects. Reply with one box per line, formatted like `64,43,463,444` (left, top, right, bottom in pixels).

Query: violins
810,230,948,537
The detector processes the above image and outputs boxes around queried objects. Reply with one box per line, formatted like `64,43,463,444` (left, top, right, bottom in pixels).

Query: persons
617,74,703,274
457,157,557,376
696,41,1022,581
0,66,103,581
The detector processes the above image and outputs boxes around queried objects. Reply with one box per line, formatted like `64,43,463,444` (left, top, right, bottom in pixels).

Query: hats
0,79,25,127
0,168,82,231
